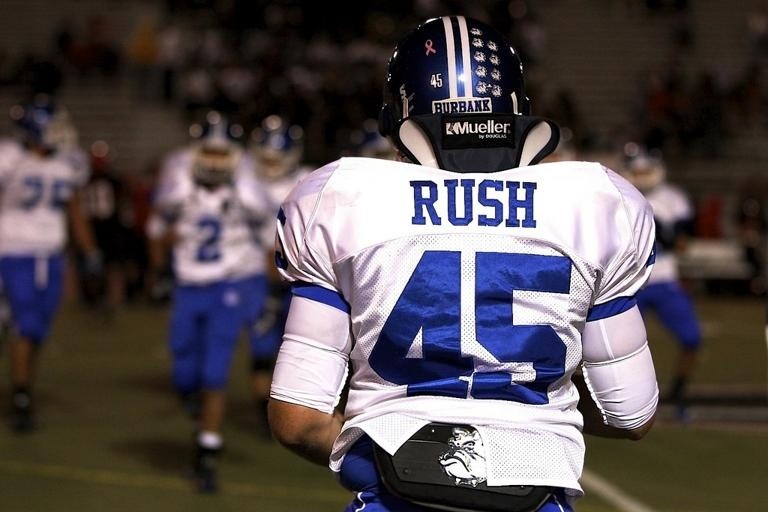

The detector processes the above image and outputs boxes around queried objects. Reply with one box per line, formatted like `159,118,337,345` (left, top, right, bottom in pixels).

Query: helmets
192,110,242,184
378,13,560,174
349,116,393,161
11,93,69,153
249,117,306,184
621,142,669,190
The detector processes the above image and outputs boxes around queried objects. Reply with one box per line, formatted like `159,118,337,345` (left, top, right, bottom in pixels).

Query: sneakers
192,429,226,495
6,384,34,435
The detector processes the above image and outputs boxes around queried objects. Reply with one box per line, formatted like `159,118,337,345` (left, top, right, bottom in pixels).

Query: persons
1,2,766,338
268,8,661,512
233,109,314,438
0,93,108,433
613,133,702,418
144,104,267,497
